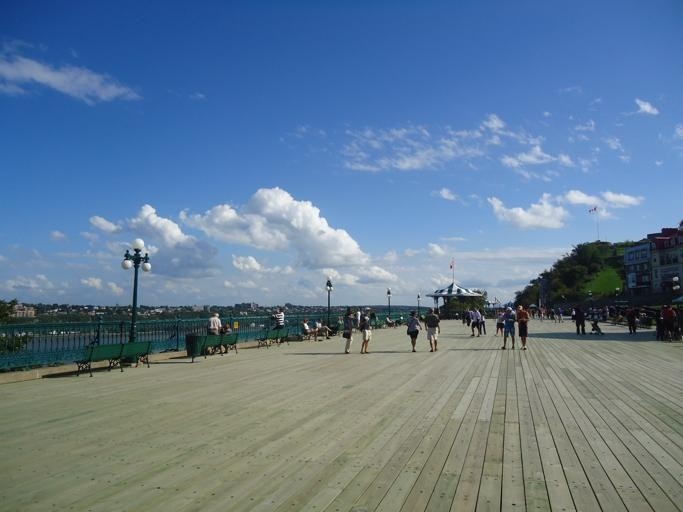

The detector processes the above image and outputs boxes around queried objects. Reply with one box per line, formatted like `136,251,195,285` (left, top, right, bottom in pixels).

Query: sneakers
345,351,350,353
430,348,437,352
360,351,368,354
412,349,416,352
501,345,527,350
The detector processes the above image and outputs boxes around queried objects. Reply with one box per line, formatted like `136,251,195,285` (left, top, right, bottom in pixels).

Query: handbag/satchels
343,331,350,338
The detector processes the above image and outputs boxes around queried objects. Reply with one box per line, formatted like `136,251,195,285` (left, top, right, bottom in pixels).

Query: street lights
587,290,594,321
417,292,422,318
122,238,151,342
325,276,334,337
615,287,620,317
671,276,680,330
386,288,391,320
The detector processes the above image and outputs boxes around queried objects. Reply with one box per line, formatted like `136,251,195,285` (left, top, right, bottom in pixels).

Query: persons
343,310,358,354
271,308,284,343
359,307,373,354
218,323,231,354
463,308,487,337
571,306,683,344
407,308,441,352
495,306,530,350
528,306,564,323
205,312,221,336
302,315,404,341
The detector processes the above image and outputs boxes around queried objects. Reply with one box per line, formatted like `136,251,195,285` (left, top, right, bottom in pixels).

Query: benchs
255,327,290,349
73,341,151,376
188,333,239,363
